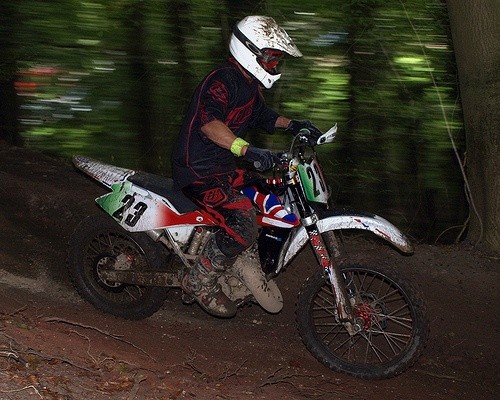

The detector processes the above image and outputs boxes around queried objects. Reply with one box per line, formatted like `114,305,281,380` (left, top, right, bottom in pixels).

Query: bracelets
230,137,249,156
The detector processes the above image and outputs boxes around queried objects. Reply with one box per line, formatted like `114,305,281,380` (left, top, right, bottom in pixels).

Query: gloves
243,147,281,172
284,120,325,143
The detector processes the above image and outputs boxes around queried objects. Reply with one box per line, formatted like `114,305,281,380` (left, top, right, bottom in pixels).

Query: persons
173,16,325,318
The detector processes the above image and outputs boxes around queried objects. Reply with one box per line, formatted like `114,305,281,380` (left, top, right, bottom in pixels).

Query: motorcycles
66,125,431,380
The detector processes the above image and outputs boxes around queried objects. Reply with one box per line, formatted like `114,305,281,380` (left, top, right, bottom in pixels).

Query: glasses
259,51,284,72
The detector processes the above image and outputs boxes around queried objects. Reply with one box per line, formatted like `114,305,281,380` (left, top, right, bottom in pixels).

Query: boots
182,234,238,318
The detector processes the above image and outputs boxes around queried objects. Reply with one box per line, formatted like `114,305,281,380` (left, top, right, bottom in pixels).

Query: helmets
229,16,303,89
